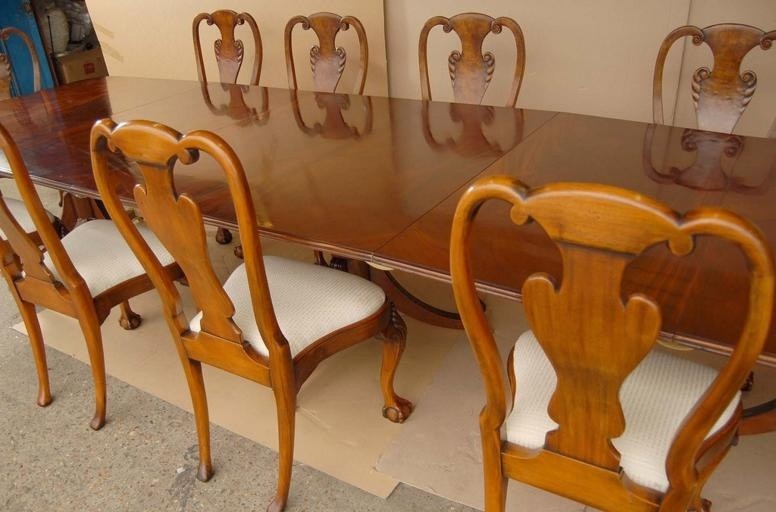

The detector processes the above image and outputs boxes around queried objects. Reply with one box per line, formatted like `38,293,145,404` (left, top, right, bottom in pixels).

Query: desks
0,77,559,331
375,112,775,416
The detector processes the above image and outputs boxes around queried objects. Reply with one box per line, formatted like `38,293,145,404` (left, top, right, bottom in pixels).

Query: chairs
90,118,414,511
192,9,264,88
0,119,190,432
449,174,775,512
418,12,527,108
284,12,369,94
0,27,41,100
651,23,776,138
